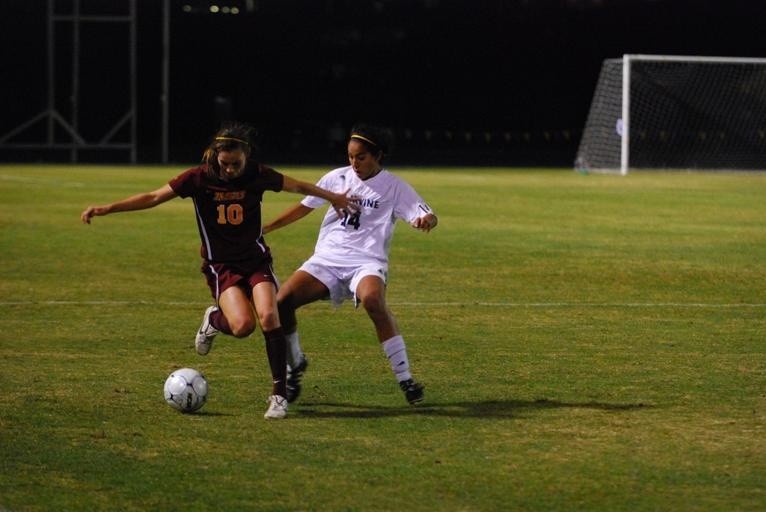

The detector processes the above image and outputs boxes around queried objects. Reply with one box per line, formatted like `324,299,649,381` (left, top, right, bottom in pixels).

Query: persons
79,124,361,419
264,125,438,404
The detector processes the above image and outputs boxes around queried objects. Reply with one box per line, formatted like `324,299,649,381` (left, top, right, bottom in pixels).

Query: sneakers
400,379,425,404
264,354,308,420
195,305,219,355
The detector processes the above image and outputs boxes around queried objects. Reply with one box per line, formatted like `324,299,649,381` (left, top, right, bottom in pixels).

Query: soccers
164,367,208,413
